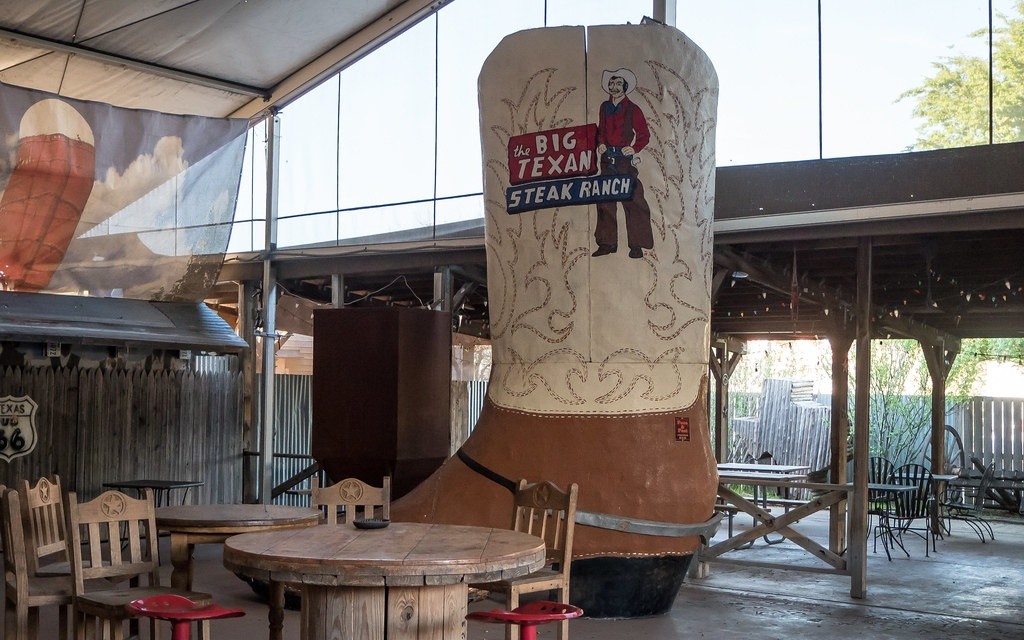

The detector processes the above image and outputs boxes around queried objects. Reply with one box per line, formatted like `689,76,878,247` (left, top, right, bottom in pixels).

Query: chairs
311,475,390,524
467,478,579,640
0,474,213,640
868,457,996,557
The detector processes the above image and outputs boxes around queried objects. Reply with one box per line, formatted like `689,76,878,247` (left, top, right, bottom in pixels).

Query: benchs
743,497,810,505
714,504,771,512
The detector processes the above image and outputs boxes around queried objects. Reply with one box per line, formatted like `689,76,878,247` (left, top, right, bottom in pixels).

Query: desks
223,523,546,640
840,483,919,561
717,463,811,550
889,472,958,540
144,504,324,590
103,480,204,566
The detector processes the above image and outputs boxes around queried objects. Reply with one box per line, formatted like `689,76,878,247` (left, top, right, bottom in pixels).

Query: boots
238,15,724,621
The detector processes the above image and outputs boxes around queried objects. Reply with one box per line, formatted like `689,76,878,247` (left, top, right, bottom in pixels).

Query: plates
352,518,391,529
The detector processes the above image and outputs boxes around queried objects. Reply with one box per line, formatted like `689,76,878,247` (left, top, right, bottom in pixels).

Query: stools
127,594,246,640
464,600,583,639
